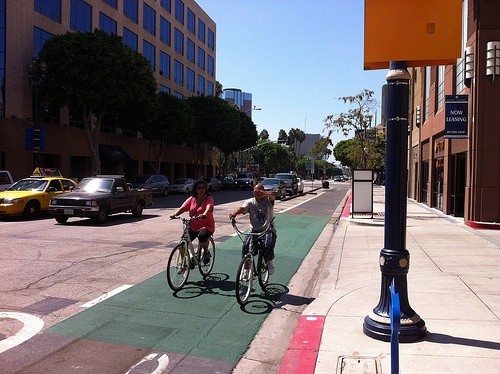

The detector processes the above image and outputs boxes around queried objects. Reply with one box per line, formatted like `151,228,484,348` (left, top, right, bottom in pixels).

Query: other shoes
202,250,211,264
266,261,275,275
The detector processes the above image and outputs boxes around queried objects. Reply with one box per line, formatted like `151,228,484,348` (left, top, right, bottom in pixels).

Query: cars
334,175,349,182
169,178,195,195
217,176,234,184
297,178,304,193
233,173,259,190
0,176,80,221
260,178,287,199
131,175,169,198
205,178,222,192
0,171,15,192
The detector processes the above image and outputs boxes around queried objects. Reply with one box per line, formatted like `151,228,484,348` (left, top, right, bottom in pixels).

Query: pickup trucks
49,177,151,224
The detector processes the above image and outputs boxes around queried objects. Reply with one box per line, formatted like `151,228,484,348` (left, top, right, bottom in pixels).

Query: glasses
197,186,205,190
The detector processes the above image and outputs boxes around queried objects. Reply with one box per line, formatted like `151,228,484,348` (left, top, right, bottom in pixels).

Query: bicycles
230,215,276,307
166,215,216,293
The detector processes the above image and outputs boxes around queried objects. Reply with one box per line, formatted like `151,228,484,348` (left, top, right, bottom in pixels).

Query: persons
230,184,277,278
170,181,215,274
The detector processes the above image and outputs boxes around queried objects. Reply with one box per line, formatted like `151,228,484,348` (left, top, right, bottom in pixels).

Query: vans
276,172,298,197
